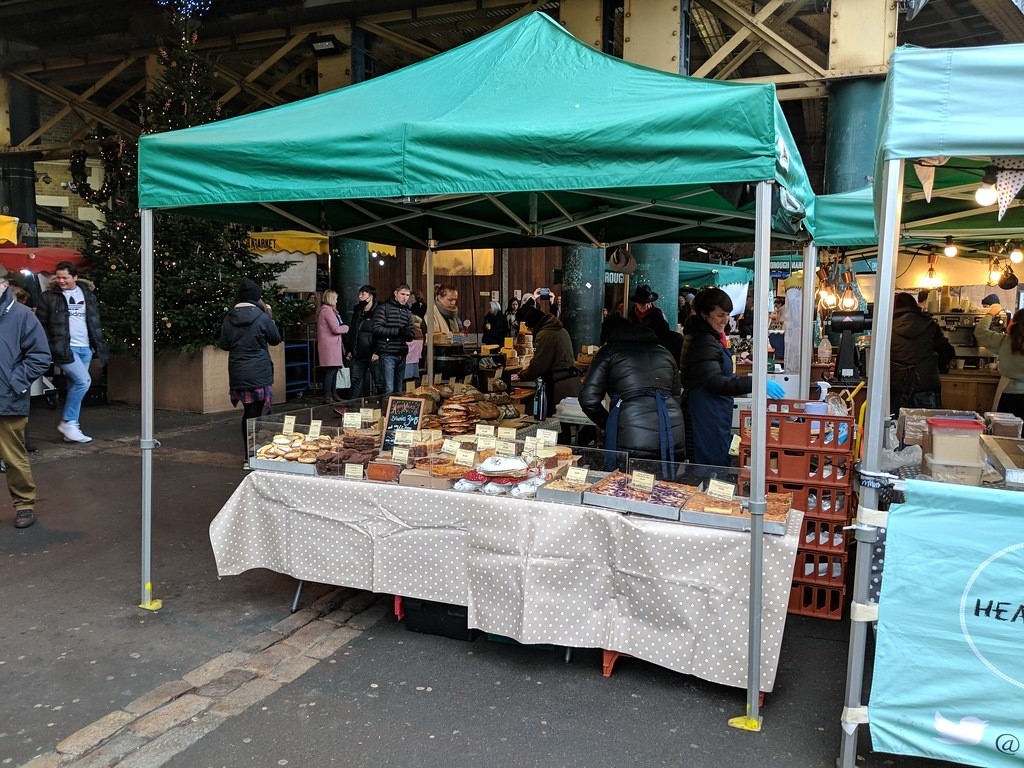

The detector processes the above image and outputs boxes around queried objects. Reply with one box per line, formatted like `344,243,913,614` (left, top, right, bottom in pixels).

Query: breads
258,380,792,523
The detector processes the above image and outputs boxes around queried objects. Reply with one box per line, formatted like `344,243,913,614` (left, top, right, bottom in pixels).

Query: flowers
278,298,316,331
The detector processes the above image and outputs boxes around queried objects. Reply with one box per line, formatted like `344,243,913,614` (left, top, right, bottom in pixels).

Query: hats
0,266,8,278
239,277,261,302
982,294,1000,305
628,285,658,303
600,315,631,347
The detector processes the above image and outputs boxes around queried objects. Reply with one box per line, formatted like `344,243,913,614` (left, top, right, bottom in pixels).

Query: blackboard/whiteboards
380,396,425,452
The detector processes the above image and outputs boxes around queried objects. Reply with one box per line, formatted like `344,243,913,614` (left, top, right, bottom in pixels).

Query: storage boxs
896,408,1024,486
250,456,793,535
555,404,587,417
738,399,855,620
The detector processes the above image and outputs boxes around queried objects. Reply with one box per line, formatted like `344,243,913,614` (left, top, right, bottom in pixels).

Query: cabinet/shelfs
285,327,310,399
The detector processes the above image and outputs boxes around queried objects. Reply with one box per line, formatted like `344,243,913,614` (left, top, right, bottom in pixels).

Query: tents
816,43,1024,768
137,11,816,724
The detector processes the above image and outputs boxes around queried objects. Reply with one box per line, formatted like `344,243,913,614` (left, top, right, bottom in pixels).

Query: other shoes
324,396,335,403
24,442,40,452
0,455,7,471
243,461,251,469
332,395,342,402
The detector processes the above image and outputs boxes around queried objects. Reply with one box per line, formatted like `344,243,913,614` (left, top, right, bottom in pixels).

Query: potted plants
78,0,286,414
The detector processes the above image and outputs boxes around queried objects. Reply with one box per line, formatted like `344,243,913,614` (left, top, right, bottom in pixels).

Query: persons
333,285,380,416
578,315,686,482
372,283,415,416
517,309,580,411
678,294,695,325
481,288,561,354
410,283,463,375
774,298,785,323
219,278,281,470
982,294,1013,318
317,289,349,404
890,292,956,420
0,264,51,527
974,303,1024,419
680,288,786,490
35,260,103,444
629,284,664,328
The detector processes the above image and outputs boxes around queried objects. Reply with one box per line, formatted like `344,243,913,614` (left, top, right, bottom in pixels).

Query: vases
287,329,306,339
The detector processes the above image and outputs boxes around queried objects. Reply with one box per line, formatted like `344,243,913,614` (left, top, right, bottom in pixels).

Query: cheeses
480,322,533,370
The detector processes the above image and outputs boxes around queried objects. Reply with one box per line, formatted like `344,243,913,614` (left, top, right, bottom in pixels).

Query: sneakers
57,420,92,442
15,509,33,528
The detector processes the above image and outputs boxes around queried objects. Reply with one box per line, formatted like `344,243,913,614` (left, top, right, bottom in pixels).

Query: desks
515,416,562,439
286,339,319,396
210,469,805,706
552,413,740,447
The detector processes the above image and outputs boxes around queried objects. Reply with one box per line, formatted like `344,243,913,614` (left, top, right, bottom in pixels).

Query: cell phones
540,288,550,295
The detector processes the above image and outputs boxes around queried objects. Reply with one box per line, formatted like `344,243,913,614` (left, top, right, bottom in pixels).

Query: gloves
766,379,785,401
399,325,410,336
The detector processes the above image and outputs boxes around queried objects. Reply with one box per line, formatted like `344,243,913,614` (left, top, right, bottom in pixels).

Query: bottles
533,378,548,421
818,335,832,363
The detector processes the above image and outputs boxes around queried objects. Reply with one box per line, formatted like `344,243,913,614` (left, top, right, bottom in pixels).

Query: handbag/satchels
890,361,921,420
335,361,351,388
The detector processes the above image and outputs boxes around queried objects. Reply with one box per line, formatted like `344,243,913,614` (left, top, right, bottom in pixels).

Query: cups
805,402,828,429
957,360,964,369
513,388,521,395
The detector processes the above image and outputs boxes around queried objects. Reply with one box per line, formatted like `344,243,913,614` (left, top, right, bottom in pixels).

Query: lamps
998,270,1019,290
306,34,350,57
